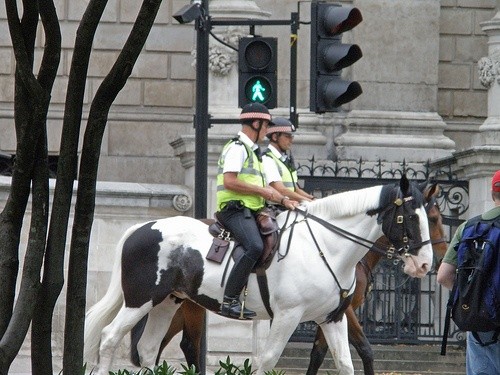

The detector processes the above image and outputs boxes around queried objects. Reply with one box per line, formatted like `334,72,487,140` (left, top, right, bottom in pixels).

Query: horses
130,184,447,374
83,172,433,375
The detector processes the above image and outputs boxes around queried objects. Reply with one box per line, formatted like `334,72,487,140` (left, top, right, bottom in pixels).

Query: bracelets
281,196,290,205
311,196,317,200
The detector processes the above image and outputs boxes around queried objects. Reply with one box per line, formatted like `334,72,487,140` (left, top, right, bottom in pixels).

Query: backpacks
451,213,500,332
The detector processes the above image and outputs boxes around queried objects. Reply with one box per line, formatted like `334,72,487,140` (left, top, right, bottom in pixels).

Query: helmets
266,116,297,135
239,102,276,126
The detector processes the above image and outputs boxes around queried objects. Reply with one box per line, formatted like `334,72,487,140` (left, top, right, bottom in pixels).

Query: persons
215,102,301,319
436,169,500,375
261,117,317,216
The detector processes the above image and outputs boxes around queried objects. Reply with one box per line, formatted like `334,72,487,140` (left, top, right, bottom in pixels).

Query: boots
221,253,257,318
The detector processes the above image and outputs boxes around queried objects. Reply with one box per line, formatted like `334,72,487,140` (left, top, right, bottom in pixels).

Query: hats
491,170,500,192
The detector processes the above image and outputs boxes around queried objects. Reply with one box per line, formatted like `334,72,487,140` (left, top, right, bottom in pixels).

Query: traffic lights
237,35,281,113
307,0,367,115
